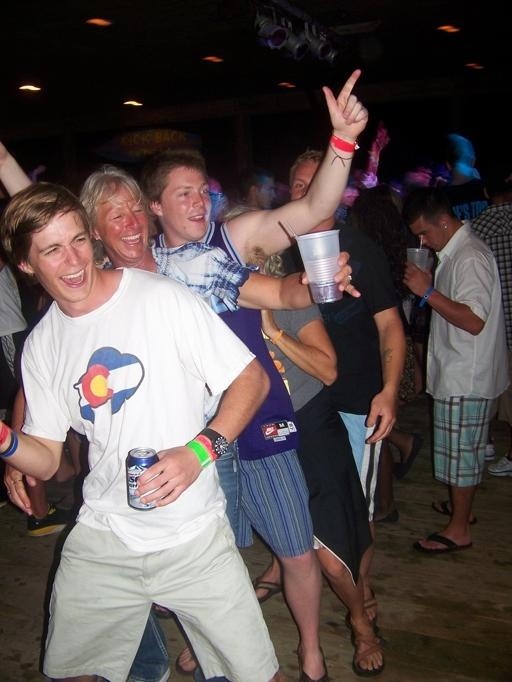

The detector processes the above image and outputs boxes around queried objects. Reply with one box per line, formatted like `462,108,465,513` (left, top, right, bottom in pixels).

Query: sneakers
484,441,496,462
297,646,330,682
26,501,69,538
485,454,512,477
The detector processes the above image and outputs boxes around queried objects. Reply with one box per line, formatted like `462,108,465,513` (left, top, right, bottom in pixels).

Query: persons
0,65,510,681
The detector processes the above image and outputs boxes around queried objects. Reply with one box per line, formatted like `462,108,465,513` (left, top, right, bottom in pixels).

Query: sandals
251,577,282,604
346,588,378,633
345,621,386,678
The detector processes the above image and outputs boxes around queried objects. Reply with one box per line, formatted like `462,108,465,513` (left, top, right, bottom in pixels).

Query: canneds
126,447,160,510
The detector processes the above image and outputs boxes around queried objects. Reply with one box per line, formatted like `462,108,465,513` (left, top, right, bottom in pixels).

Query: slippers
431,500,478,524
412,531,473,555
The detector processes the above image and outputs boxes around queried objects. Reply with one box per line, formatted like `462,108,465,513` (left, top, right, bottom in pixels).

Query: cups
407,248,430,269
294,230,344,306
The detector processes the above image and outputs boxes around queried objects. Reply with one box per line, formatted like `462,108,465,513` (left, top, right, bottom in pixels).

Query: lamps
254,0,341,64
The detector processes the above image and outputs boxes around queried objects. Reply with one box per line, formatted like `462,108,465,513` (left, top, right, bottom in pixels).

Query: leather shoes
373,509,400,524
396,431,424,482
